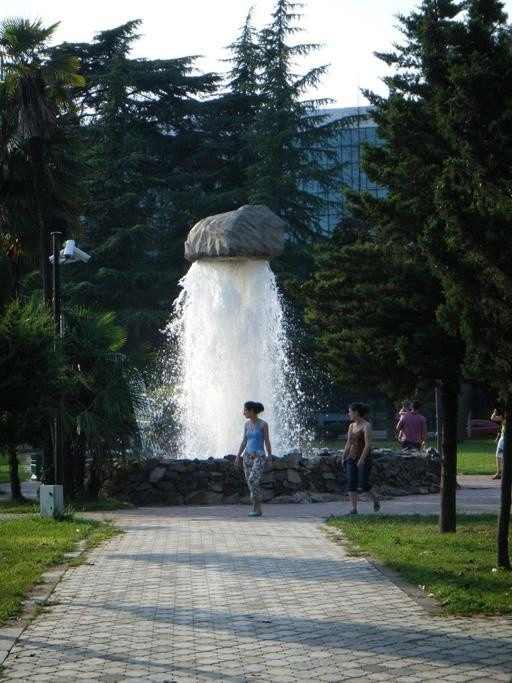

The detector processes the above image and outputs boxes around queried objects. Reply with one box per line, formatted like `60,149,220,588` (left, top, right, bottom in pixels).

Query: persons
233,399,273,517
341,402,380,515
489,405,507,480
395,399,429,452
397,398,412,442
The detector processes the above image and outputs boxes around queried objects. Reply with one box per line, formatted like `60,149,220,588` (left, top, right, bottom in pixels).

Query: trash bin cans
39,485,64,520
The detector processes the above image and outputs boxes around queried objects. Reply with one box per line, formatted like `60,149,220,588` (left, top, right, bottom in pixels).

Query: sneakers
373,497,380,512
346,508,357,513
248,511,262,516
493,474,500,479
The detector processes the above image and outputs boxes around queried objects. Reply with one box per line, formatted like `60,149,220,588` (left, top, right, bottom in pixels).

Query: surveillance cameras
48,239,91,265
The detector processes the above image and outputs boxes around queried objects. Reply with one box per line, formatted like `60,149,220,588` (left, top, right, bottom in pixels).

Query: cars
434,419,501,440
